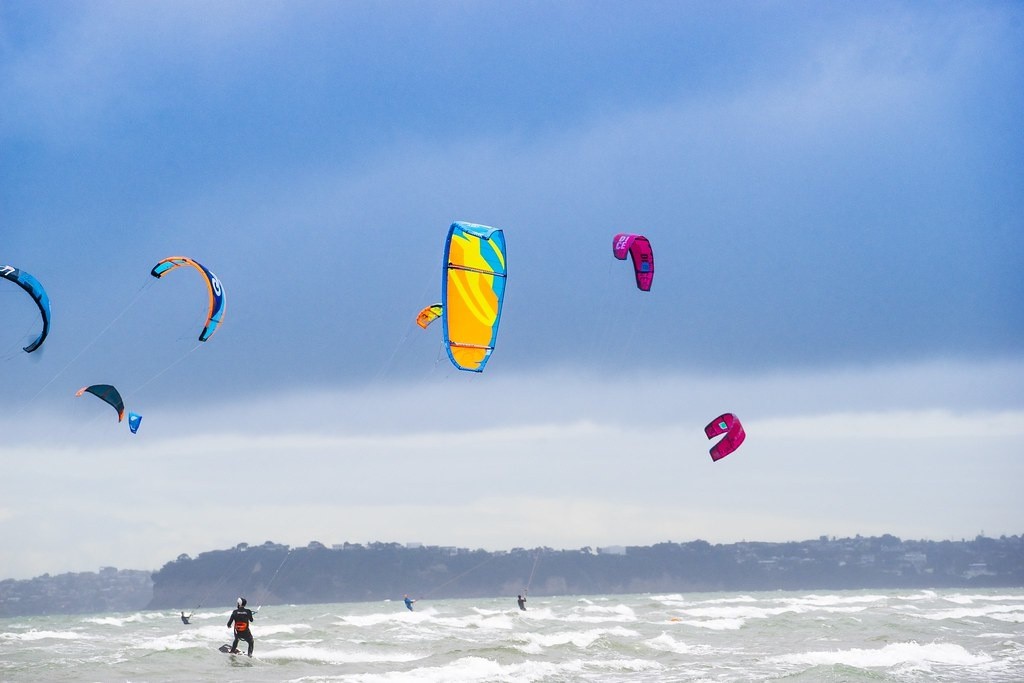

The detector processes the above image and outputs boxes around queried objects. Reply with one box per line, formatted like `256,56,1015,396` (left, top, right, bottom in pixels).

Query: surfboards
219,644,261,662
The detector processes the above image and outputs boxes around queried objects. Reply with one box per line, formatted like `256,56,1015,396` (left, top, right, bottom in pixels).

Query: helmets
237,598,247,606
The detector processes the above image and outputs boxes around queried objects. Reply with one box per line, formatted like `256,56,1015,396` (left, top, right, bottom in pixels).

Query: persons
517,595,526,611
404,594,415,612
227,596,258,657
181,612,189,624
